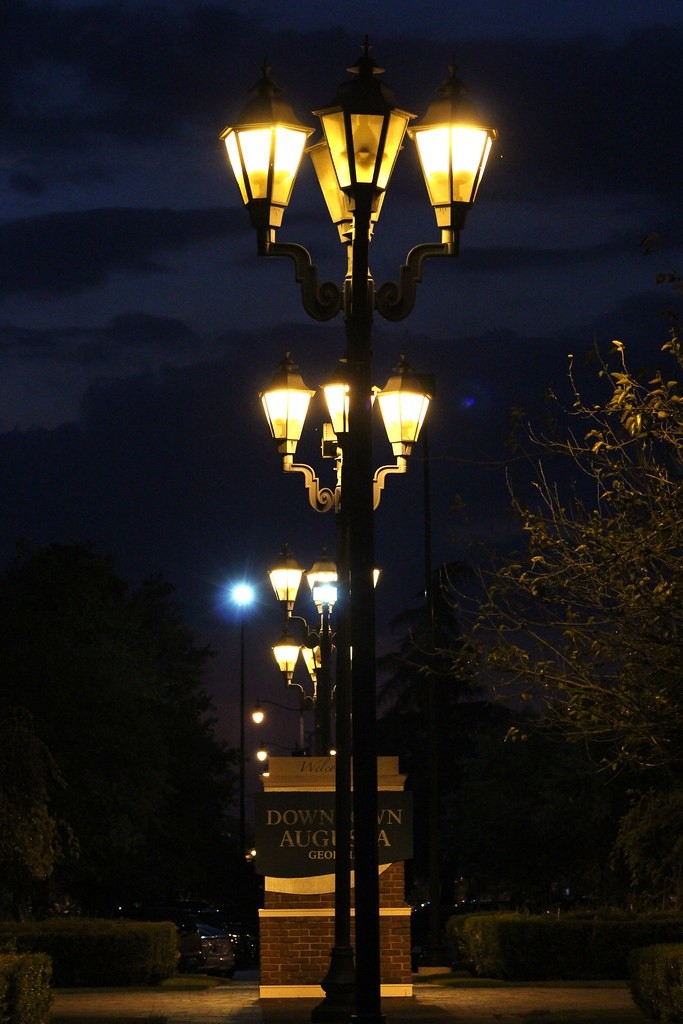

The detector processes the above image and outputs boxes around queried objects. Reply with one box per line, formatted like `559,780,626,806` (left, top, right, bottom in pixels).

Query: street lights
221,96,498,1024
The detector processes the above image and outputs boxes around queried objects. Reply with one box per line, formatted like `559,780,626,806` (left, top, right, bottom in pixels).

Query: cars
178,922,254,977
